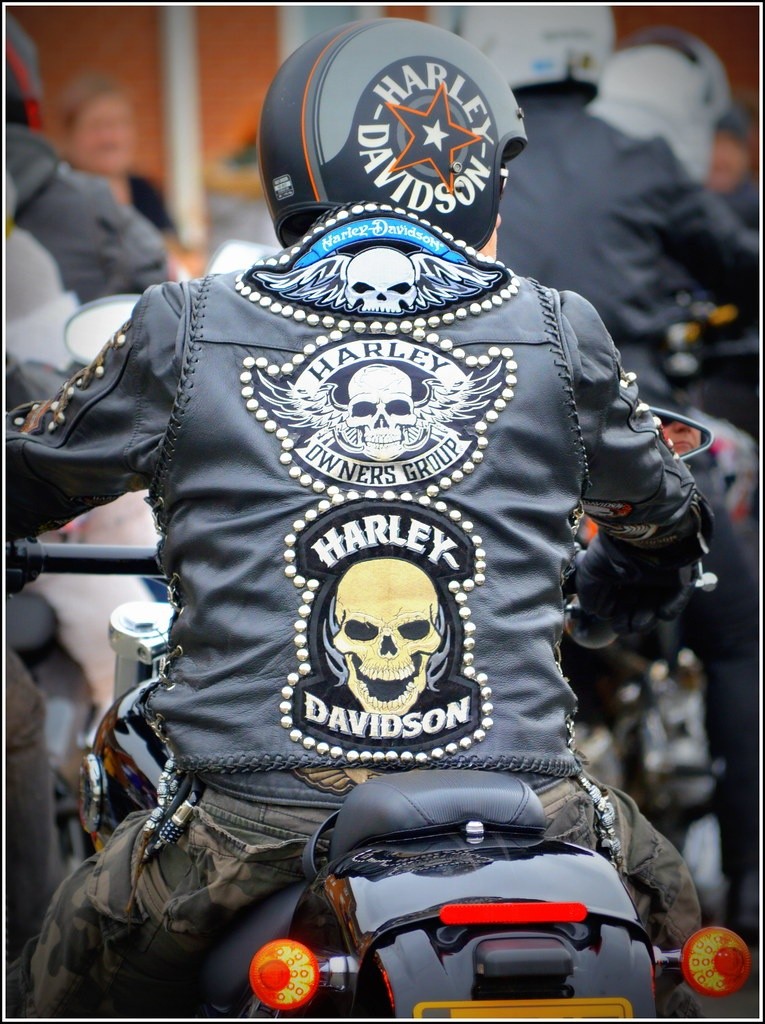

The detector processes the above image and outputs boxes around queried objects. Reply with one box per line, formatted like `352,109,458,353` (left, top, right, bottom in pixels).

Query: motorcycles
2,296,751,1020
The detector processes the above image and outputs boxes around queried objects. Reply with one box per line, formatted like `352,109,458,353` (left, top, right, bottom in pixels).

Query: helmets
258,17,528,253
6,10,41,133
587,25,732,182
460,7,615,104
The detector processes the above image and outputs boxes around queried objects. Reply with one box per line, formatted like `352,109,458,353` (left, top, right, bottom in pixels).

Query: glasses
657,415,674,426
500,163,508,200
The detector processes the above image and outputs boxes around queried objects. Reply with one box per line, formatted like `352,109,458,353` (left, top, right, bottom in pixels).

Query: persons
654,413,701,456
5,6,760,1019
